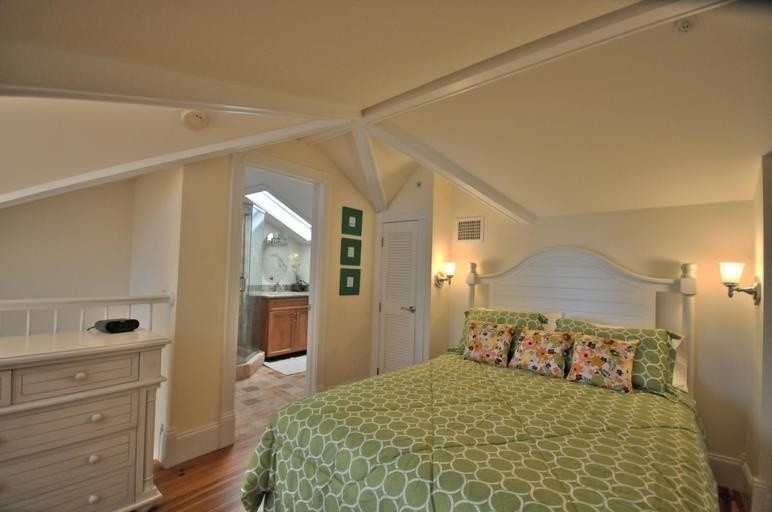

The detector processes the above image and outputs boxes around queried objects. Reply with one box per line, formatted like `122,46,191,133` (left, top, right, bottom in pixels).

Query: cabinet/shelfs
0,329,163,511
259,298,307,358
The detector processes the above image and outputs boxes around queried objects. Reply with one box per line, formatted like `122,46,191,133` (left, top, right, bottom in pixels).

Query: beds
241,247,720,512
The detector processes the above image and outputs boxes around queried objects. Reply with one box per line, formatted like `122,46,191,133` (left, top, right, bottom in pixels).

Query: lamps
434,262,455,288
718,262,761,306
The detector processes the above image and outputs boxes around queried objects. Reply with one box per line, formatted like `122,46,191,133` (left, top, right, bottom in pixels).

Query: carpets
263,355,306,375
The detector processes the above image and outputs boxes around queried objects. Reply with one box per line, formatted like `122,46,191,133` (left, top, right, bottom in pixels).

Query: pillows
461,320,518,367
565,333,641,395
551,318,680,399
455,310,548,363
507,329,582,380
